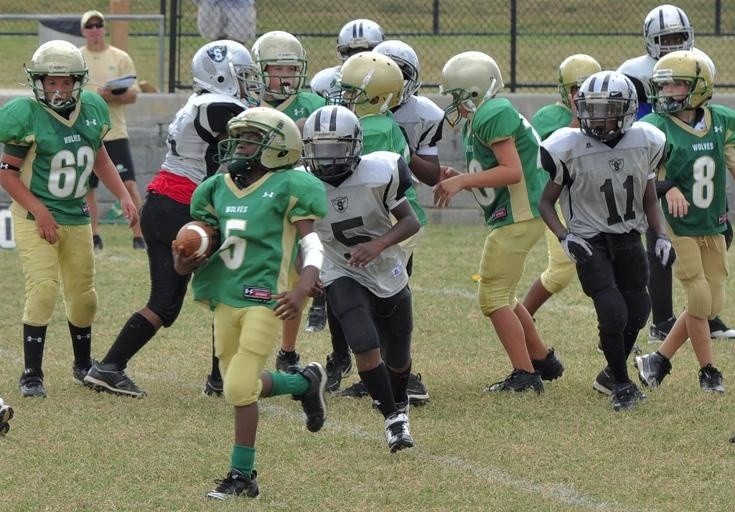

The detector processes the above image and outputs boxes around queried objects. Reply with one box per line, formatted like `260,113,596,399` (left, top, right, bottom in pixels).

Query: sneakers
595,341,643,354
340,382,368,400
201,374,224,398
401,374,431,406
697,366,725,392
276,348,300,374
483,372,545,396
0,398,14,437
92,236,104,250
528,347,564,379
132,237,144,250
708,317,735,340
304,306,327,333
383,409,414,454
19,369,48,399
591,368,631,397
634,351,672,388
85,365,148,398
206,467,261,502
290,361,326,432
648,314,677,340
72,364,91,385
325,361,349,397
609,382,647,411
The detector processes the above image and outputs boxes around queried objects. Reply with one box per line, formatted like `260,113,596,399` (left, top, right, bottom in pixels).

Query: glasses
79,22,106,31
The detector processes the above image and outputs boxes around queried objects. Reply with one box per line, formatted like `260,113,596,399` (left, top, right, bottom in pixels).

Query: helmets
303,106,363,179
559,54,602,107
190,40,265,100
577,70,640,142
337,52,402,117
337,19,383,57
652,51,716,113
23,40,88,111
373,40,421,102
217,106,303,174
439,52,504,127
642,6,694,56
252,31,309,100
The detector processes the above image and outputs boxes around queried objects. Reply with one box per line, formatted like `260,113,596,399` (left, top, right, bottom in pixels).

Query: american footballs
176,222,220,261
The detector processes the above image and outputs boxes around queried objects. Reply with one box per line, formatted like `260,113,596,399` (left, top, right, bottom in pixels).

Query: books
105,76,137,91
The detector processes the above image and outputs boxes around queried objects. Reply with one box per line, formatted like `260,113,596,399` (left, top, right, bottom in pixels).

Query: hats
80,9,104,30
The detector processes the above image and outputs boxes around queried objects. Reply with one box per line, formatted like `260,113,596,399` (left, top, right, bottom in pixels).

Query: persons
616,5,735,345
250,32,324,378
288,99,422,457
0,40,139,399
170,107,328,504
522,54,601,316
82,39,257,401
633,48,735,399
536,69,676,413
305,19,383,337
77,9,145,254
323,49,433,406
368,39,444,187
431,51,565,398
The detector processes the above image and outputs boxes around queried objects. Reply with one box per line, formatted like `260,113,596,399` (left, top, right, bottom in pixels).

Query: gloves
657,234,677,267
561,234,593,266
